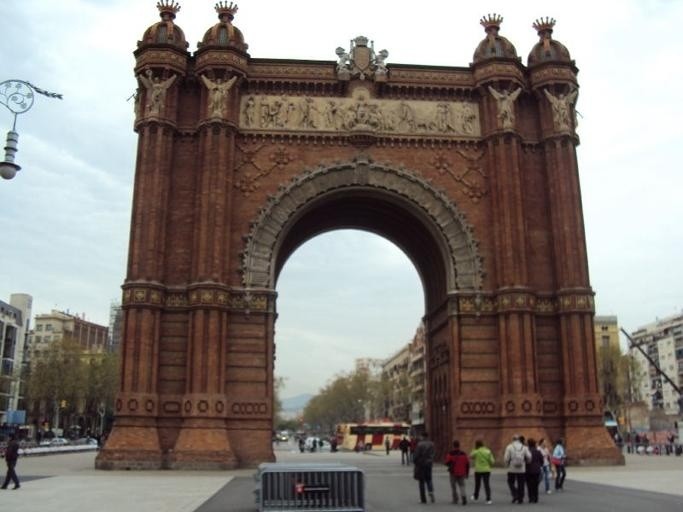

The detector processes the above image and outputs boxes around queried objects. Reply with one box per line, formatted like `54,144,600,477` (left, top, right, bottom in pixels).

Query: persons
243,96,255,129
140,71,177,113
544,89,577,130
298,434,566,505
614,430,675,455
488,86,520,130
299,98,319,130
0,422,22,489
419,102,475,131
260,94,294,129
202,75,236,116
325,97,415,130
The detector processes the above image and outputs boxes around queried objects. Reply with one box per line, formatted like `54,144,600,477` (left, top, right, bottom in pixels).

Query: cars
77,437,97,446
40,438,68,446
275,430,322,451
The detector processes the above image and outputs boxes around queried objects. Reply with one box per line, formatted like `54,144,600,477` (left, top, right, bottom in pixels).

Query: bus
335,422,411,451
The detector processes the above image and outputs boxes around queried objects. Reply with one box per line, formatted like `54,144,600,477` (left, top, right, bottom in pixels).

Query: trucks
0,425,36,456
604,409,624,448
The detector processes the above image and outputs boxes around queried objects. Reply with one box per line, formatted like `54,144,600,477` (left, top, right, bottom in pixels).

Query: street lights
0,73,64,183
